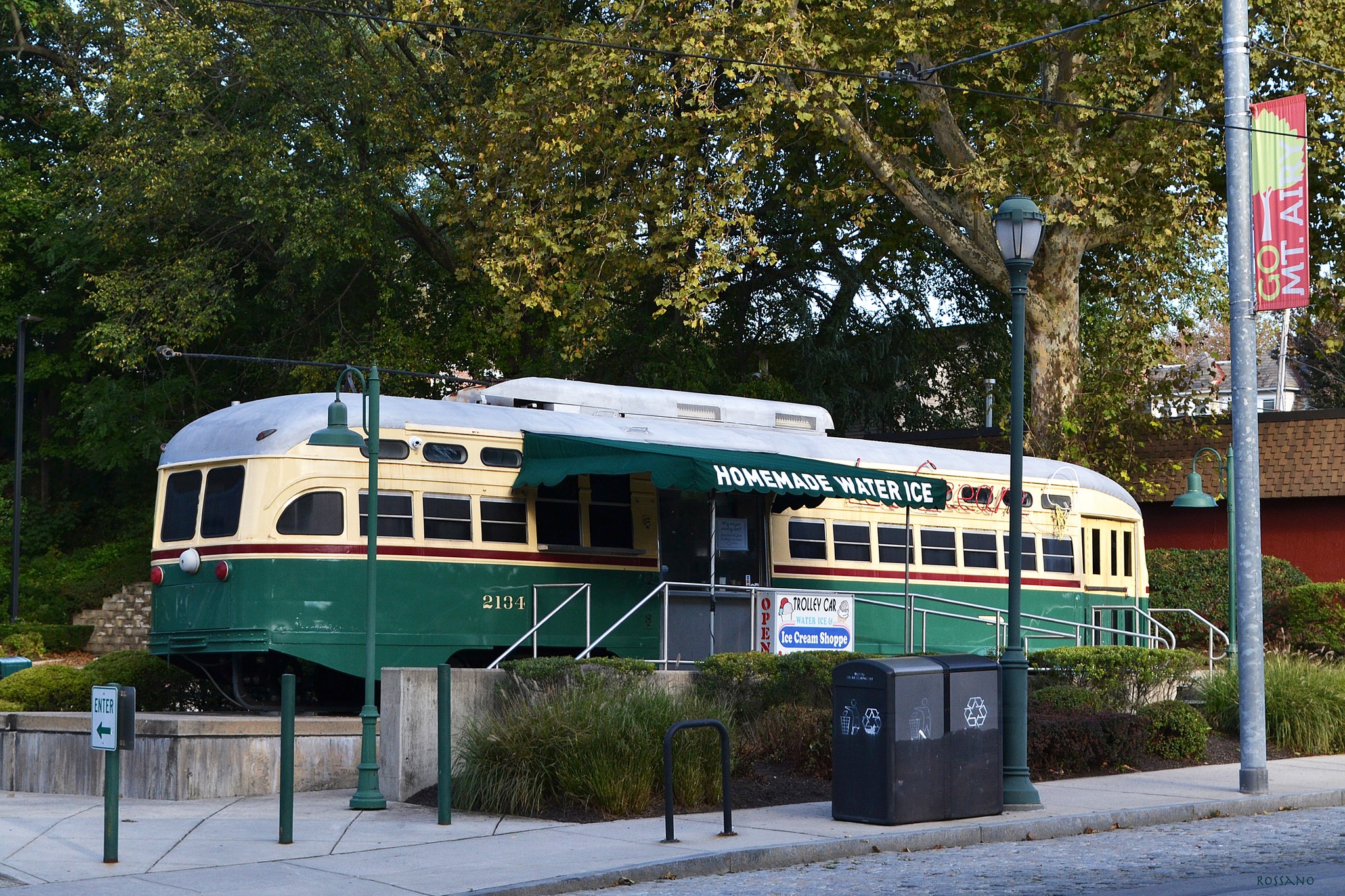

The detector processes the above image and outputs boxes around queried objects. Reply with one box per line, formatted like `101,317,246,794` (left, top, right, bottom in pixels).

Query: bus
147,344,1152,714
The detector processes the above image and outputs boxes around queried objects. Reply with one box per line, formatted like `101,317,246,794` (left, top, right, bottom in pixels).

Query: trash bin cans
831,654,1003,826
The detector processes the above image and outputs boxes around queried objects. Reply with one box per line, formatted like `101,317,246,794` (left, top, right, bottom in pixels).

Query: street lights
307,361,389,811
11,314,44,622
995,183,1044,808
1170,443,1238,675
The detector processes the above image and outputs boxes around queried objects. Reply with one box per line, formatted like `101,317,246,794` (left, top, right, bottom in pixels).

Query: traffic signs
91,693,117,752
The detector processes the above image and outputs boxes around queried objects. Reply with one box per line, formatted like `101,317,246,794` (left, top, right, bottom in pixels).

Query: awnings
510,430,947,655
